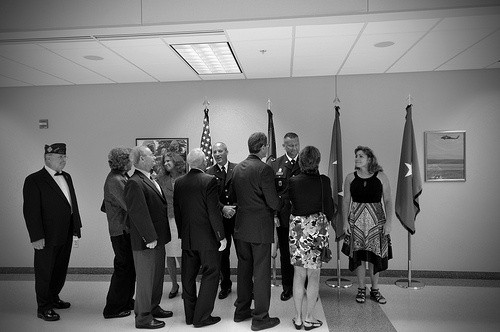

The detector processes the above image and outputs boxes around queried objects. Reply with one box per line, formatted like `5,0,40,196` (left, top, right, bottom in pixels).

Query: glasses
261,143,269,147
164,160,171,163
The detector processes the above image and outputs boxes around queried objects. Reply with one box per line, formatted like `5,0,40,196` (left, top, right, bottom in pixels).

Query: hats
45,143,66,154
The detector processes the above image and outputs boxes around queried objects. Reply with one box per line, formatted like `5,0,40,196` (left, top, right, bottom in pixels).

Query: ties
150,175,156,187
222,167,227,178
290,160,295,167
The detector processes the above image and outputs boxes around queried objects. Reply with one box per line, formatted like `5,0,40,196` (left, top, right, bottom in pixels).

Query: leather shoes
49,300,70,308
102,310,131,318
38,309,59,321
219,287,231,299
151,309,172,317
136,318,165,328
126,299,136,309
281,288,292,301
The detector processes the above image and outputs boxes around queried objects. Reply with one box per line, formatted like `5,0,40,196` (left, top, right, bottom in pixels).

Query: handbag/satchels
321,247,332,263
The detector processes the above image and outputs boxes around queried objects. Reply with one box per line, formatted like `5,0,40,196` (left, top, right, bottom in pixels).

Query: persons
103,146,139,319
22,142,83,321
205,142,254,300
124,146,173,330
158,152,189,300
342,146,393,304
287,145,337,329
232,133,286,331
173,148,228,328
269,132,319,301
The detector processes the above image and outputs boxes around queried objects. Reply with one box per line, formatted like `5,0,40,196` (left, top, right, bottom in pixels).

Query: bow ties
54,172,63,176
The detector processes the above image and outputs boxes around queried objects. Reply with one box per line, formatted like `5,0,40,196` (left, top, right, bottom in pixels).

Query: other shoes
234,309,255,322
252,317,279,330
212,317,221,324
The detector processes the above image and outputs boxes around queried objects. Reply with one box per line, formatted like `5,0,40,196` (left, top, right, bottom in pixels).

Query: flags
199,111,215,170
326,105,349,243
394,104,423,235
264,108,278,168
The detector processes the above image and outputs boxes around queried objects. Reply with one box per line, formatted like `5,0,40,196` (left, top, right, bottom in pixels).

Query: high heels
169,284,179,298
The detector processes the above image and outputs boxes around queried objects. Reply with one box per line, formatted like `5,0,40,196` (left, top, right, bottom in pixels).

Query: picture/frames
425,130,467,182
136,137,189,219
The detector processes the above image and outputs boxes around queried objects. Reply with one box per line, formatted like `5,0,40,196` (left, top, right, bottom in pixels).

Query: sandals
304,319,322,329
370,287,386,303
292,318,303,329
356,287,366,303
